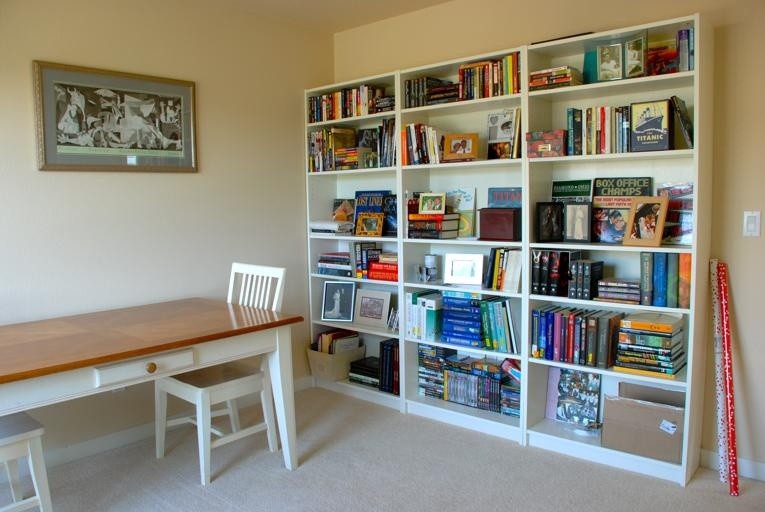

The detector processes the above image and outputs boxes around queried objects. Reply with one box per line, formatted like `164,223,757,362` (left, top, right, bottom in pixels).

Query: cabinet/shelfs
399,46,525,447
525,11,714,486
305,70,398,411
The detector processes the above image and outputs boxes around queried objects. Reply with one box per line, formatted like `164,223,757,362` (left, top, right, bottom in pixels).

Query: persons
631,204,660,239
452,140,470,153
593,210,626,241
574,207,584,240
628,42,641,74
328,289,348,314
600,54,619,79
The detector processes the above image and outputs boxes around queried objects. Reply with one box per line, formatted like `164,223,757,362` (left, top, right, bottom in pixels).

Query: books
567,96,693,155
405,247,522,354
405,187,476,239
401,123,444,165
531,305,687,380
531,250,691,310
316,241,398,281
592,177,652,208
552,180,591,203
310,190,397,237
556,369,601,430
404,52,519,109
535,201,565,242
315,331,400,396
417,345,520,418
656,187,693,246
308,86,397,172
528,65,583,91
487,108,521,160
676,28,695,71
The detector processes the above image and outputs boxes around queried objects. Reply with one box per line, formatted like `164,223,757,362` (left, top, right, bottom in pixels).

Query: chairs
1,411,58,512
154,260,306,487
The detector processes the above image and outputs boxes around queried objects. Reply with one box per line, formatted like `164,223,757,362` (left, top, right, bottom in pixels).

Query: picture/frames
31,55,199,175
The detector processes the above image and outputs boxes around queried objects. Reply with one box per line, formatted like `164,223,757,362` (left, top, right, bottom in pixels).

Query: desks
1,295,304,473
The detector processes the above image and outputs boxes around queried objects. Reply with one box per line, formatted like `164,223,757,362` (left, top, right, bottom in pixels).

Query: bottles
423,254,438,283
